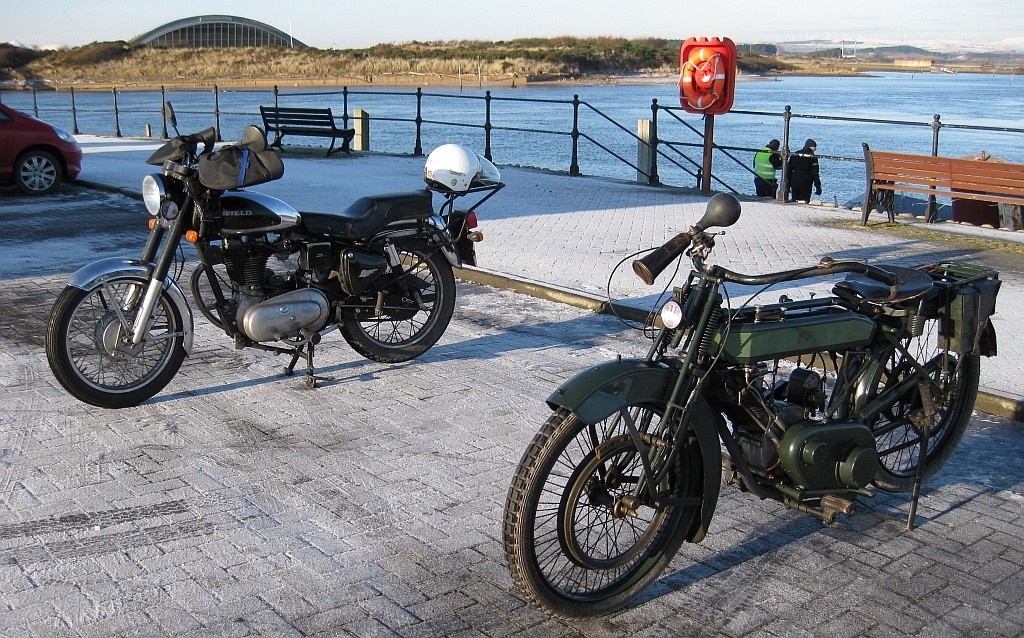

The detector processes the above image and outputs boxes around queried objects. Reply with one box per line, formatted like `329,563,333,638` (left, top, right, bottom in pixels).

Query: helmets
423,143,489,197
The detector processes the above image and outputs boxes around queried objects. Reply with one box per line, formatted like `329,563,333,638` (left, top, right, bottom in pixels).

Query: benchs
860,143,1024,226
260,106,356,156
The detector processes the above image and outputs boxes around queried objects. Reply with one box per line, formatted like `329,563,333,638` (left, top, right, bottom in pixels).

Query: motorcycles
43,99,503,411
501,192,1002,626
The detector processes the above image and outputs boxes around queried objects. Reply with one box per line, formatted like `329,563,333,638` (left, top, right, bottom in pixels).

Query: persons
753,139,782,198
788,139,822,203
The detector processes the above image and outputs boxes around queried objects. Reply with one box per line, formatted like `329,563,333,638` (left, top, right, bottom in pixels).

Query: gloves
814,187,822,195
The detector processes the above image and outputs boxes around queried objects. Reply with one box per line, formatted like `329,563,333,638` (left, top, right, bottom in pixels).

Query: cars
0,100,82,197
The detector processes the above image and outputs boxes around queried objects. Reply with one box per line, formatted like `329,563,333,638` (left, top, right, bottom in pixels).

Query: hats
805,139,817,148
769,139,780,148
